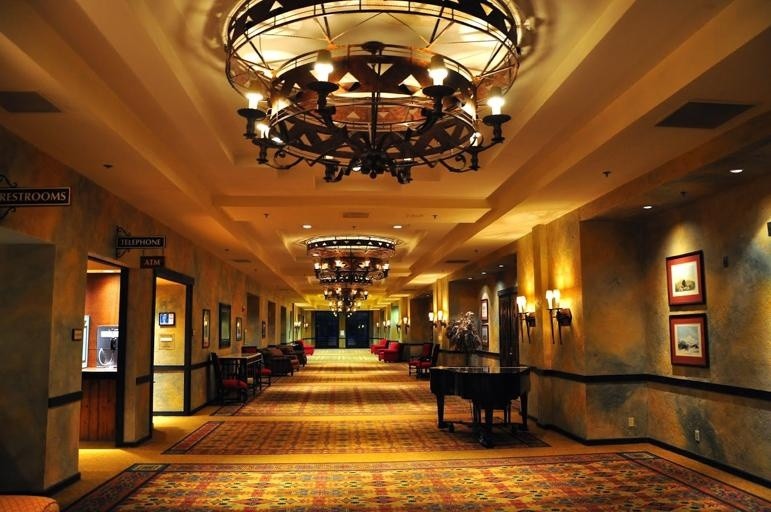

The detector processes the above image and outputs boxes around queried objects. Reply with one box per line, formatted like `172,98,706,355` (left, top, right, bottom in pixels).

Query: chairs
371,339,440,378
211,340,315,405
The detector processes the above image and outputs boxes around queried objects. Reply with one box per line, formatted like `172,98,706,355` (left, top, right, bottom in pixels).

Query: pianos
429,366,530,442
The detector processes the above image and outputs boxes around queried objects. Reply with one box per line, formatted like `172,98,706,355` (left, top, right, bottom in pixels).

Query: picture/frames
236,317,243,341
159,312,175,326
665,250,706,307
480,325,489,349
481,299,488,321
219,303,231,348
203,309,210,348
668,316,706,369
261,320,267,337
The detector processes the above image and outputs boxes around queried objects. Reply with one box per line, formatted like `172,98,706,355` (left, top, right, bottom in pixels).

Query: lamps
306,235,396,318
222,0,524,184
428,311,436,328
294,321,308,333
377,317,410,334
545,290,573,346
437,311,447,328
515,295,536,344
